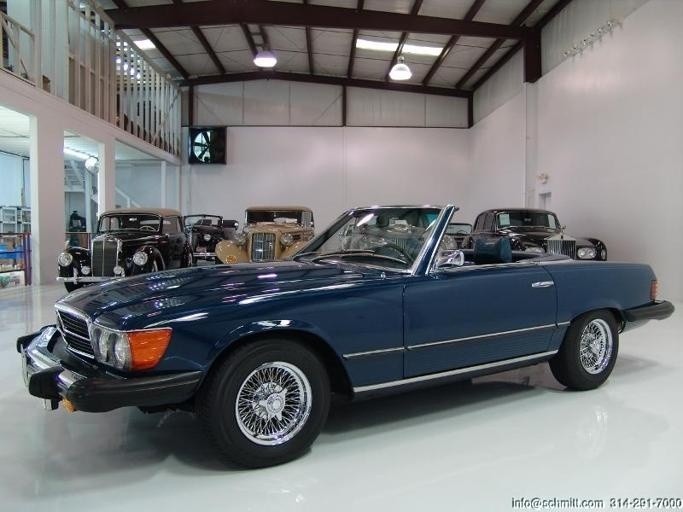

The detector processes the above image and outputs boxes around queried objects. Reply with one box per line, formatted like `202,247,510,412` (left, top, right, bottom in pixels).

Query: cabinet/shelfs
0,232,31,288
0,206,31,233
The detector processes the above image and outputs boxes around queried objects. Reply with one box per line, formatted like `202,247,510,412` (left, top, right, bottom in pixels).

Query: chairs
473,237,514,264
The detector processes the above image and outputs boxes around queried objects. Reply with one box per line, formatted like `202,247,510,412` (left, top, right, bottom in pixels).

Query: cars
461,208,608,265
16,202,674,471
56,203,316,293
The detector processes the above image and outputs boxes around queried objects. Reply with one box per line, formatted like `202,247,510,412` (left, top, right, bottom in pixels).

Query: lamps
252,46,278,69
388,53,414,83
